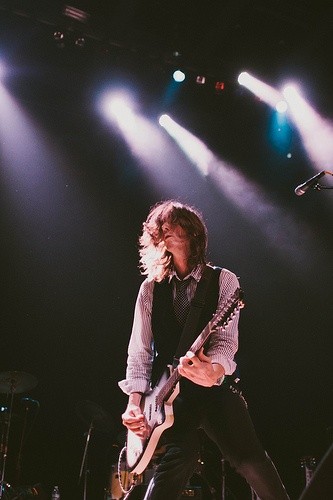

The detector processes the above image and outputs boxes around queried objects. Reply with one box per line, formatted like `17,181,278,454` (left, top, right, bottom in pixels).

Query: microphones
294,171,326,196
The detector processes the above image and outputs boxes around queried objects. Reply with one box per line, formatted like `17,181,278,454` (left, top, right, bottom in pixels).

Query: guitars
117,288,247,493
299,455,317,486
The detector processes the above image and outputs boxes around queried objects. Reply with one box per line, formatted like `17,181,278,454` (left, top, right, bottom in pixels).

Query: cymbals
0,369,38,394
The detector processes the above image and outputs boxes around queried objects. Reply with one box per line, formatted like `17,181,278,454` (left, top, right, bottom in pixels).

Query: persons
118,199,290,500
